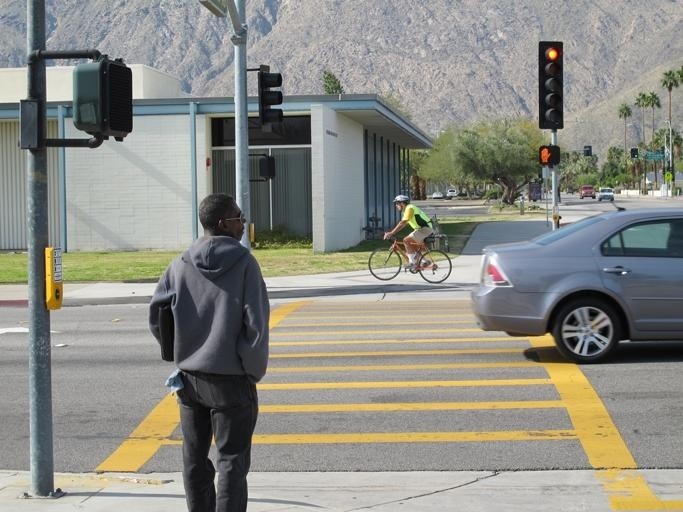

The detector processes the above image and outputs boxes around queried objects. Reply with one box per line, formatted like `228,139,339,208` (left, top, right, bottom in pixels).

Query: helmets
393,195,408,203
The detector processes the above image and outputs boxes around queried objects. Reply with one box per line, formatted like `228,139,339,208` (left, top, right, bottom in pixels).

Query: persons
146,193,270,512
383,194,434,270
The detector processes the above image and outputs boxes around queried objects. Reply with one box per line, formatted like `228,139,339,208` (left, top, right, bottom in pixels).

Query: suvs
595,187,615,202
579,184,595,199
544,186,550,194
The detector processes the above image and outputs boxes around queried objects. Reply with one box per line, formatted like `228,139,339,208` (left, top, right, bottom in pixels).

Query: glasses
223,214,245,225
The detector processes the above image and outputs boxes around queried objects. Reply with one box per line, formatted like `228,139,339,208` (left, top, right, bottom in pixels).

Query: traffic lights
104,62,134,129
543,46,560,124
266,71,284,123
264,155,276,179
631,149,634,159
634,148,638,159
540,145,556,168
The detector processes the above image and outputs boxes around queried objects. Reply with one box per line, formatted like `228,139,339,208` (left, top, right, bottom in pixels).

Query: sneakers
421,260,430,267
403,264,418,269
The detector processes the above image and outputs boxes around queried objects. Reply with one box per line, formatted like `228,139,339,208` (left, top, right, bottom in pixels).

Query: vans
566,185,573,195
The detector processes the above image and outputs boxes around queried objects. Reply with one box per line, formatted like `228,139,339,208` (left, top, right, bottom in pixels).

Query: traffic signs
645,152,665,160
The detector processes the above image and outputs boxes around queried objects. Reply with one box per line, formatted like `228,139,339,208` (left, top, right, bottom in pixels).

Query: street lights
664,120,674,190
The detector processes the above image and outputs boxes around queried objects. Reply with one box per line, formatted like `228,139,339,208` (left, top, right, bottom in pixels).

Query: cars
433,191,444,198
547,189,553,194
446,188,457,198
470,208,682,365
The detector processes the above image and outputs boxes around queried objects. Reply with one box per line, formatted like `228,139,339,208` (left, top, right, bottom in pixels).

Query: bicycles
366,231,454,286
